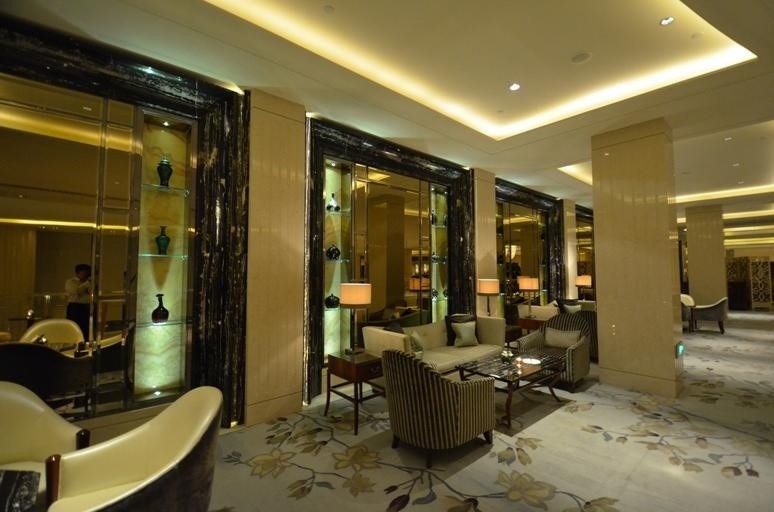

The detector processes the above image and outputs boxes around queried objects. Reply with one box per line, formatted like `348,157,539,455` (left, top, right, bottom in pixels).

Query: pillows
452,322,479,348
381,306,419,319
383,320,404,336
445,315,478,346
410,331,424,351
544,327,581,350
551,297,597,315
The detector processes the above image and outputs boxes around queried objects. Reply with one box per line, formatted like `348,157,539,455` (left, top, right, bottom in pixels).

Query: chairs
1,382,92,509
689,296,728,334
0,340,93,420
680,294,694,332
18,318,86,364
45,384,224,511
61,322,137,412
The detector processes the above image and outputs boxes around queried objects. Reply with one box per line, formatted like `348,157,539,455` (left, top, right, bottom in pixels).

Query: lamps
519,277,539,319
575,275,592,302
339,283,372,354
477,279,500,315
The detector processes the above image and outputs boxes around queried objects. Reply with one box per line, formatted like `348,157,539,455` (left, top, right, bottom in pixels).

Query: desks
323,350,388,435
382,349,497,469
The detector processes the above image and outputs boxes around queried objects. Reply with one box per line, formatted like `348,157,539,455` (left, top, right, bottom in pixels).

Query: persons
63,263,93,343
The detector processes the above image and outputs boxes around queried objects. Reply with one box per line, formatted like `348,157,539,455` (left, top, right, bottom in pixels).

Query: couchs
516,293,598,365
516,312,590,393
349,304,431,354
362,314,507,397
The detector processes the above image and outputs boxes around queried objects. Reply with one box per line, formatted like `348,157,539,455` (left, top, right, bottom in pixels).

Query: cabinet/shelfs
429,222,449,306
318,202,359,375
134,178,192,333
